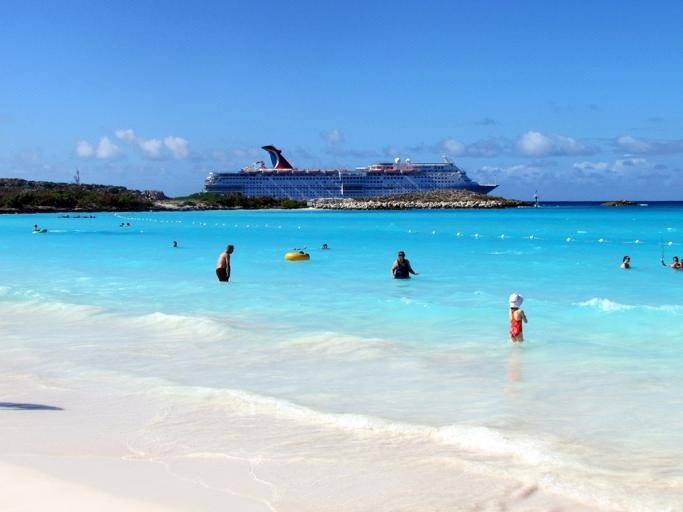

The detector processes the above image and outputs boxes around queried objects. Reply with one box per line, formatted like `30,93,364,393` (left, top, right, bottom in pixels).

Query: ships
202,145,500,202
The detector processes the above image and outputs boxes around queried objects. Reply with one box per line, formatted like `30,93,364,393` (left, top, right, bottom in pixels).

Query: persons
34,225,39,231
621,256,630,268
172,241,177,247
215,244,234,281
509,294,528,343
671,256,683,268
323,244,328,249
391,251,420,279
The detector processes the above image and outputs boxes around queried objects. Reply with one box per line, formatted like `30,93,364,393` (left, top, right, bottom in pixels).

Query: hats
509,294,523,307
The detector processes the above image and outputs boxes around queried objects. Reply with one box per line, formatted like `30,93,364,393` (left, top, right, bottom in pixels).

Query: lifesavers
285,254,309,260
